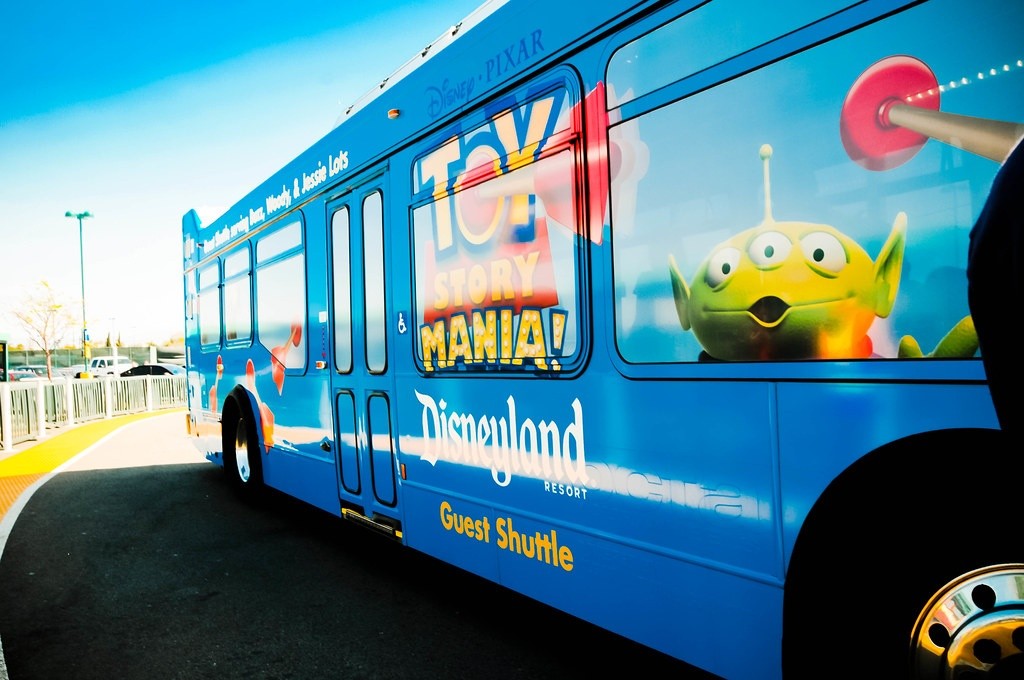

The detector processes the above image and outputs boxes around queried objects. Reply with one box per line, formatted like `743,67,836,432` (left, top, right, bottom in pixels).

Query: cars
120,363,186,377
8,365,83,382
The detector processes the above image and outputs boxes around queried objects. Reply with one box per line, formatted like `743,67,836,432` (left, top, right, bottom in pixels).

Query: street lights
64,210,94,378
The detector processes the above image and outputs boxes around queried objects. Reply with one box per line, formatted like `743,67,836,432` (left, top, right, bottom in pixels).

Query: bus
182,0,1024,680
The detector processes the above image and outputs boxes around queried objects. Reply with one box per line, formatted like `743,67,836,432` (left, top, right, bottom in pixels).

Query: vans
89,356,138,378
